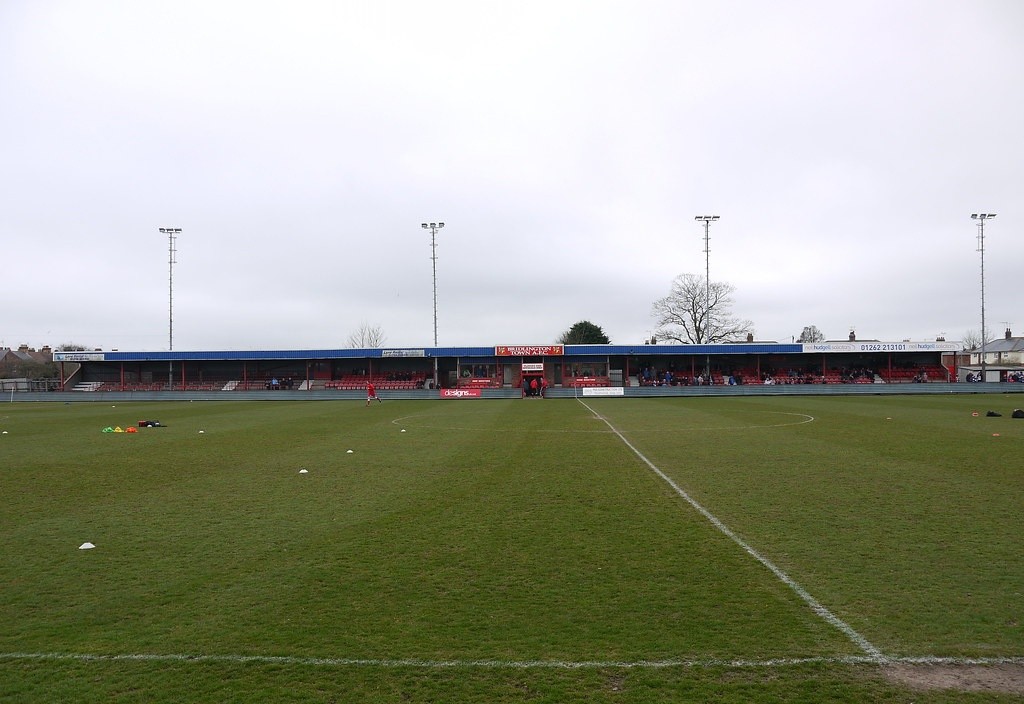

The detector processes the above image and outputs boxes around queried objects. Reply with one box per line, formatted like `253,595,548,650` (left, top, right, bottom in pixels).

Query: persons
386,370,442,391
365,380,383,407
265,379,271,389
530,377,539,397
539,377,547,397
571,365,1024,384
463,369,471,378
280,378,287,389
522,379,530,397
476,365,486,379
287,377,293,390
271,377,278,389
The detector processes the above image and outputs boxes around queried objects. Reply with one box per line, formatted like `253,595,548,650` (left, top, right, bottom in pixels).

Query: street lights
695,216,720,377
971,214,996,383
159,228,183,390
422,223,445,389
